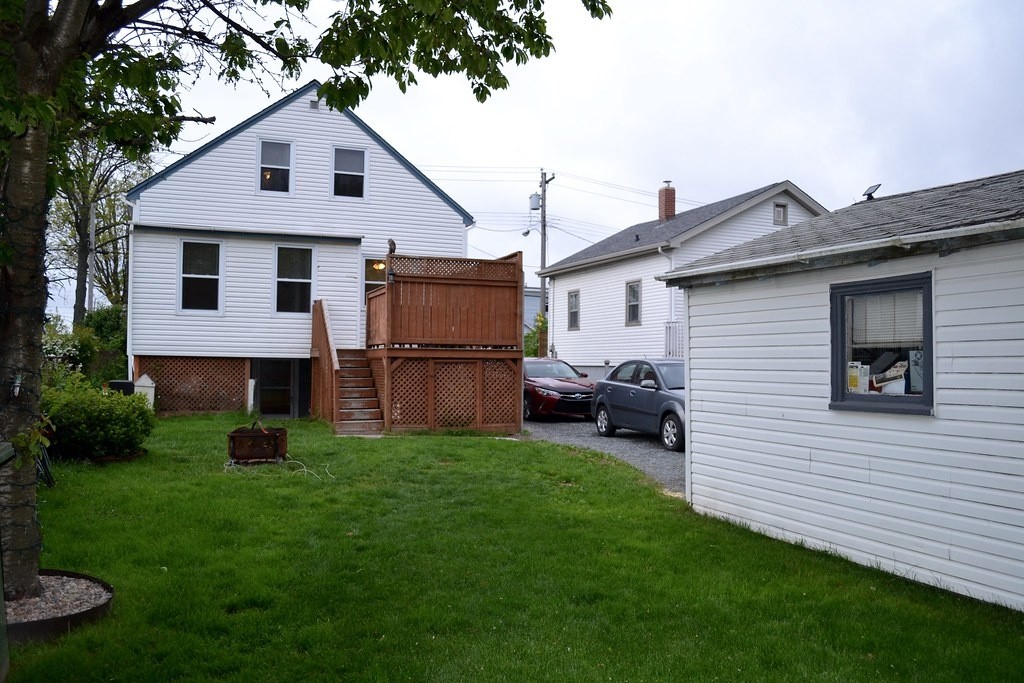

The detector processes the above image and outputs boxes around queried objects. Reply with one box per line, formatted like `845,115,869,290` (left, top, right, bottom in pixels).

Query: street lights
523,167,556,320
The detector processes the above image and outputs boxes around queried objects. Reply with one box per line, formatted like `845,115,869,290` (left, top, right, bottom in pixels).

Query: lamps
373,263,385,270
264,171,271,179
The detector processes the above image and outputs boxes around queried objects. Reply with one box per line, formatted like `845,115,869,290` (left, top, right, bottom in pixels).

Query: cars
591,358,689,454
513,354,597,426
